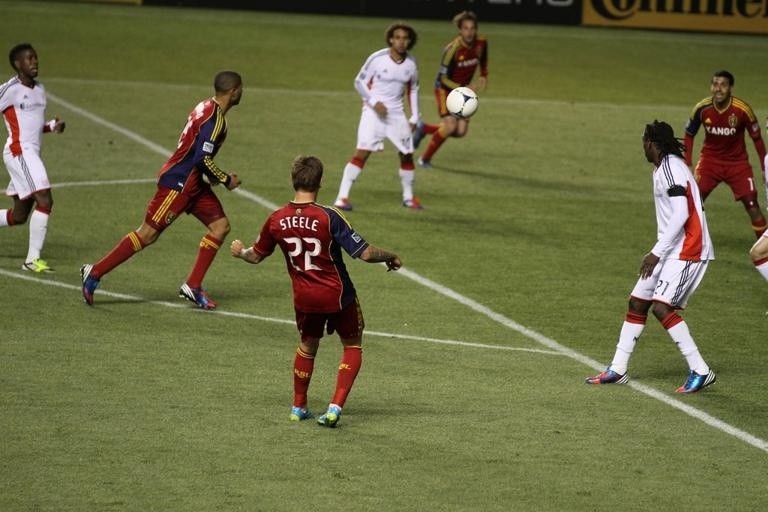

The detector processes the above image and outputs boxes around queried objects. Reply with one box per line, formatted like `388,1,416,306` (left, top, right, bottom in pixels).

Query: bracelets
366,96,378,108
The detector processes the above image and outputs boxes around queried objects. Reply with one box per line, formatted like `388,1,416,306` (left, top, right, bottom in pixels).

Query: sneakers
290,406,313,420
179,282,216,310
403,195,426,209
334,198,353,209
417,158,432,168
675,368,716,394
585,367,629,385
318,404,342,427
22,259,55,274
80,264,99,306
414,121,425,148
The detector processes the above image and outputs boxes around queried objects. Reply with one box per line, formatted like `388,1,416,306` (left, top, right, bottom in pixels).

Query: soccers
445,86,479,120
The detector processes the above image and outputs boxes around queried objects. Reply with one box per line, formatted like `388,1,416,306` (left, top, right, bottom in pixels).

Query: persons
0,43,66,274
79,71,243,311
682,70,767,240
748,117,768,315
334,23,422,211
229,155,402,428
413,10,488,169
585,119,716,394
